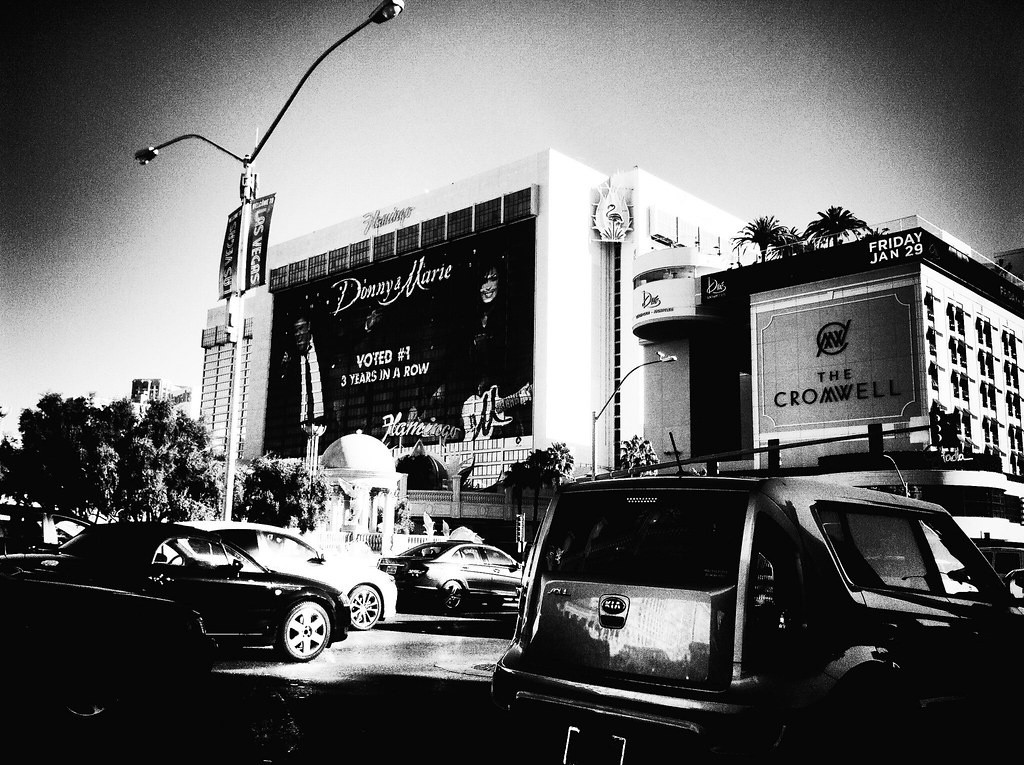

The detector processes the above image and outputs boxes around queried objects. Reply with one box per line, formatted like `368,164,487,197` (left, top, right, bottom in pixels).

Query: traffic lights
516,514,525,542
929,411,957,447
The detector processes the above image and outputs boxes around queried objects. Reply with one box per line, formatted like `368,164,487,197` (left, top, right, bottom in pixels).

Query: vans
976,546,1024,583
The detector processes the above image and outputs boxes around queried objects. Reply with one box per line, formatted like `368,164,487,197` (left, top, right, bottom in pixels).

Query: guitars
461,380,533,442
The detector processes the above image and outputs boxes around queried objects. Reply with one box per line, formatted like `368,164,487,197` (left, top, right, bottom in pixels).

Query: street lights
134,0,406,522
592,356,678,481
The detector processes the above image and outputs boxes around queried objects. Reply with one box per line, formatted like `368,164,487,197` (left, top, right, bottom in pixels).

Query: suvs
484,469,1024,765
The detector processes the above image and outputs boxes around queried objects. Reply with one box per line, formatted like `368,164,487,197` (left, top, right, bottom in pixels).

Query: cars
174,521,398,633
0,504,96,568
53,520,351,663
0,572,219,734
376,539,523,616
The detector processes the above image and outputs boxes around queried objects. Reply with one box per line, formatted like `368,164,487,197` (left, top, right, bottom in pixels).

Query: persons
282,313,339,458
451,256,534,441
